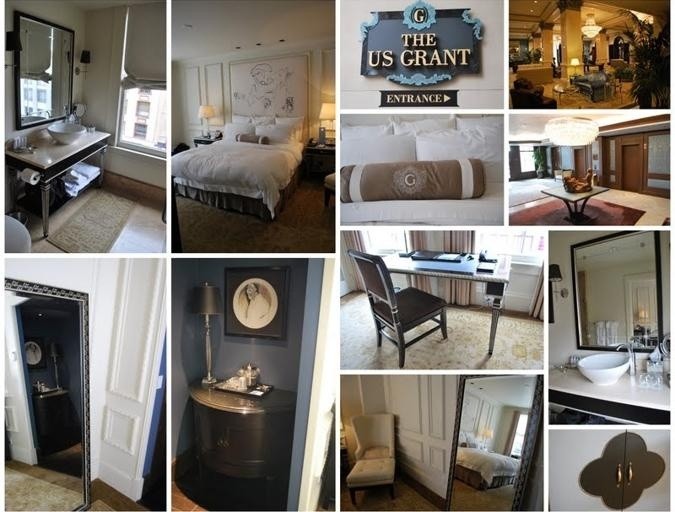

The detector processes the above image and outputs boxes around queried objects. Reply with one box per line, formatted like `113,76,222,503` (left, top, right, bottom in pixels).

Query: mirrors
73,100,87,127
570,231,664,353
13,10,74,129
443,374,544,510
4,276,92,510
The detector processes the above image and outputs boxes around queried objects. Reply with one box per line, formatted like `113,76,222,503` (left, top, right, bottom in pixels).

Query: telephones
479,249,497,263
307,136,319,147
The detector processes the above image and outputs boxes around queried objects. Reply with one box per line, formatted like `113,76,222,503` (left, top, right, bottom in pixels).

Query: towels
64,170,80,197
74,162,99,177
75,168,100,182
61,173,88,193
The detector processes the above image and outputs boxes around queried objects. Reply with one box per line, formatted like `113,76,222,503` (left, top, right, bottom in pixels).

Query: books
411,250,462,263
477,262,495,273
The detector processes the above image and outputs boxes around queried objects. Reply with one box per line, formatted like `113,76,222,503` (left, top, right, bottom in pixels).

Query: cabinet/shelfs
31,389,73,464
341,443,355,483
549,429,671,511
188,383,297,479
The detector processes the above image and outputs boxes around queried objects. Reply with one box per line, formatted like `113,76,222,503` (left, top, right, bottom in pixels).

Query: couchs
567,71,614,104
510,89,558,109
516,64,554,83
514,77,544,94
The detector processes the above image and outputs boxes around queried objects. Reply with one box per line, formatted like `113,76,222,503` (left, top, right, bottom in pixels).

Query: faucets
64,105,70,122
616,344,631,353
46,111,50,118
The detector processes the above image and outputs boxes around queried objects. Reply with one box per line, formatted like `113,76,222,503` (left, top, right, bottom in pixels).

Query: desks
584,62,606,74
8,129,111,236
542,184,610,224
549,364,670,424
381,250,513,356
634,334,658,347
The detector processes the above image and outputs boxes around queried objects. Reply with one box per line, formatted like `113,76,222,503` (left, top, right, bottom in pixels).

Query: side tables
603,78,624,105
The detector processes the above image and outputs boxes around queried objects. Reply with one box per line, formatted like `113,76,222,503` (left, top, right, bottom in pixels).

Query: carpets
42,189,136,253
510,197,646,225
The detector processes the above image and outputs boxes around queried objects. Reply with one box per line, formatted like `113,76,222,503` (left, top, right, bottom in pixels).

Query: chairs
346,414,397,505
612,58,637,83
347,249,450,367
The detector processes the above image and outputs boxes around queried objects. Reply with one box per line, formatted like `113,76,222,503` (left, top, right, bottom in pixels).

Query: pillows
390,114,456,138
340,137,416,166
416,128,503,186
251,115,276,126
235,133,271,145
231,115,251,124
340,159,485,203
456,116,503,129
340,120,394,140
255,125,290,144
458,431,488,453
223,123,255,141
275,116,303,143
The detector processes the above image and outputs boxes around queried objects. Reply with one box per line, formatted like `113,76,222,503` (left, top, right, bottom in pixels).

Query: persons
584,167,593,184
245,283,269,324
26,344,38,365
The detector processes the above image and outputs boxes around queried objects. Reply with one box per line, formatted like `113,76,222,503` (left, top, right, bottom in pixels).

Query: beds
170,114,306,224
453,430,522,491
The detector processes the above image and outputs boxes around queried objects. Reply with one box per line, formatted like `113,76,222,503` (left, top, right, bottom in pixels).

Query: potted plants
532,147,547,178
512,49,521,73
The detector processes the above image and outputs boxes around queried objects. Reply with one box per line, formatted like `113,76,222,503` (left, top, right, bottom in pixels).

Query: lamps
319,103,336,146
43,341,65,391
189,278,224,385
76,51,91,80
543,117,601,148
480,426,494,451
550,264,569,297
637,305,649,335
340,414,351,447
197,104,217,139
5,31,23,68
580,14,603,40
570,58,580,74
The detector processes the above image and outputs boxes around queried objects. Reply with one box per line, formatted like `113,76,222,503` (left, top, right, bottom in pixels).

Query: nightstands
192,135,221,147
302,138,334,179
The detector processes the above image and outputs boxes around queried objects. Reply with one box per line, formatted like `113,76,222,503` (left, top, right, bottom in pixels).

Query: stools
322,171,335,208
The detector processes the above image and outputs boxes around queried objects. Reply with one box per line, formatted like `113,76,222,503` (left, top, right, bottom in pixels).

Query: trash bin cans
6,209,33,236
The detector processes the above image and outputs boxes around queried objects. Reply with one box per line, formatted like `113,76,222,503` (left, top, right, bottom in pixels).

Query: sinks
21,116,46,126
47,123,86,144
577,353,629,385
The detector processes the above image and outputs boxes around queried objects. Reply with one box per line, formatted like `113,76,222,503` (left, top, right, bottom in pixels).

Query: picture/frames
223,266,290,341
20,334,46,369
